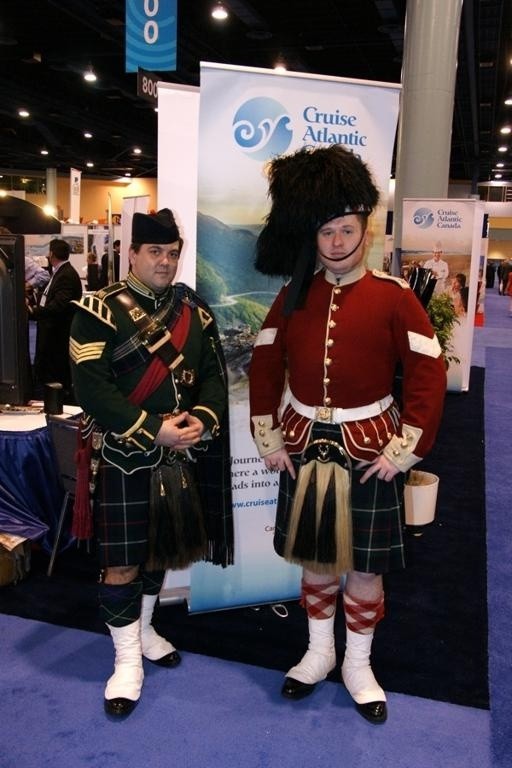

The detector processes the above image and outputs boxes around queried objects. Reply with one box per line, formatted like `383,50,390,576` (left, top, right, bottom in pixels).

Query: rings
270,464,278,471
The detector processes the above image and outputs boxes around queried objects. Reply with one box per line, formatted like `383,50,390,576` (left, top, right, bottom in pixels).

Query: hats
433,240,442,252
132,209,180,245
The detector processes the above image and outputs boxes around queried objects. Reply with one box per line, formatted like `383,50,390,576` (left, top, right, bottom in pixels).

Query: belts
286,388,393,425
152,413,182,427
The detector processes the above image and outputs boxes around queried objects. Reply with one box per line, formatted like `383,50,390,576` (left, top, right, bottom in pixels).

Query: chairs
45,412,94,578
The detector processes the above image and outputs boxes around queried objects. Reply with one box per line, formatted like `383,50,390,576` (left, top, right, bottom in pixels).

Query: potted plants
401,292,463,527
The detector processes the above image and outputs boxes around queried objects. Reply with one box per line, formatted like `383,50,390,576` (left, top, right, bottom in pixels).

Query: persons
87,252,99,291
99,238,122,288
423,251,449,295
24,239,83,400
25,255,51,304
440,286,469,391
443,273,466,302
485,257,511,296
66,208,229,720
247,211,447,727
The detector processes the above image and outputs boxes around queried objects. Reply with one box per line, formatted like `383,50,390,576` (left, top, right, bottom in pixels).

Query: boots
341,624,388,724
105,614,146,717
282,611,338,700
140,594,180,669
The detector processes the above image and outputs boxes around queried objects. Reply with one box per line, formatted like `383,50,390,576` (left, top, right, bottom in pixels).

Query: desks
0,398,85,573
